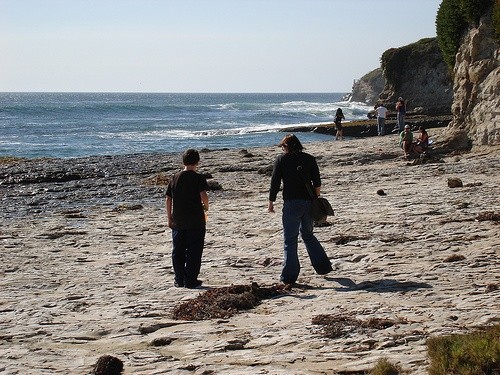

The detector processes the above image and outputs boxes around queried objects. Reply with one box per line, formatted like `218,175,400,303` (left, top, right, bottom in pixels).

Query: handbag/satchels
312,198,334,221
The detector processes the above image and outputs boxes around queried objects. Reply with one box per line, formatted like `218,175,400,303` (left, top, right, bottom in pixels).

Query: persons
417,126,428,154
165,149,209,289
400,125,413,158
268,133,334,285
375,103,388,136
335,108,345,140
396,97,406,133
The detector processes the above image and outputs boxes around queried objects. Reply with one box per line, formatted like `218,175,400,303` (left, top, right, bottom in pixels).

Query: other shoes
185,280,202,288
174,279,184,287
280,276,295,285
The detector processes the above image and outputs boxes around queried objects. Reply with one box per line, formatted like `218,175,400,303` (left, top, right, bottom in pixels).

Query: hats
405,124,410,129
